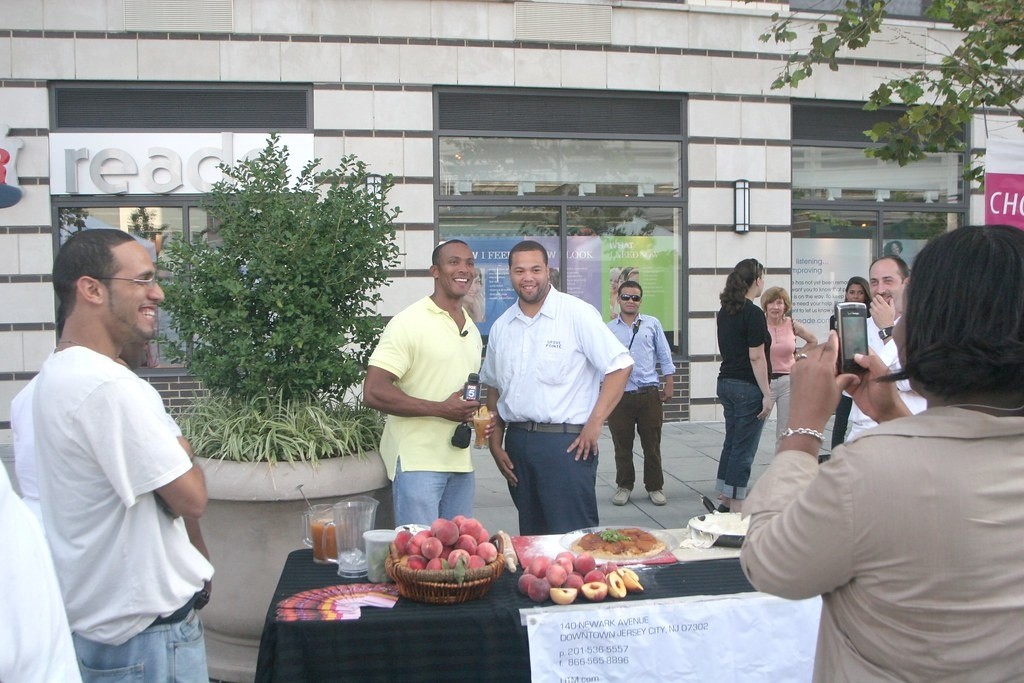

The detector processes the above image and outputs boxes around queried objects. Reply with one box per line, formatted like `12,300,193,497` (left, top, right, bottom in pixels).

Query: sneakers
612,488,631,505
648,490,666,506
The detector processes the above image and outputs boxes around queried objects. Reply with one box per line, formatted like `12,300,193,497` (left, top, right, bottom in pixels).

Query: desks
253,527,824,683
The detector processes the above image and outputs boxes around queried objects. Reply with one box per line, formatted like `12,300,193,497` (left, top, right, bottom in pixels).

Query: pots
688,494,751,548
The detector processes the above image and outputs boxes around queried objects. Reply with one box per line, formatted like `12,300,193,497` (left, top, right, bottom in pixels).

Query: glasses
619,294,642,301
93,277,157,288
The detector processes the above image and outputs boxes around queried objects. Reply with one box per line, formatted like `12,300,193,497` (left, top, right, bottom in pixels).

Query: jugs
321,496,379,579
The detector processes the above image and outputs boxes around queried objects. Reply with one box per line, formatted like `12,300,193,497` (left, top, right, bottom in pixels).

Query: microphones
452,373,480,448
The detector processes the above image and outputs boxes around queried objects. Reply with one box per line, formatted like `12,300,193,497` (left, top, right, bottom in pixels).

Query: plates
557,524,677,562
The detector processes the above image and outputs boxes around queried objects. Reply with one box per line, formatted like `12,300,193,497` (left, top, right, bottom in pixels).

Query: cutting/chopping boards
510,534,677,569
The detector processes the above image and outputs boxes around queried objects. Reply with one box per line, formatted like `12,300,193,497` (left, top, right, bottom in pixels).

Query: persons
715,258,773,514
463,268,486,322
828,276,872,452
609,267,639,319
0,227,214,683
760,287,818,451
361,237,499,529
738,224,1024,683
476,239,635,537
884,240,904,255
601,280,676,506
844,254,928,443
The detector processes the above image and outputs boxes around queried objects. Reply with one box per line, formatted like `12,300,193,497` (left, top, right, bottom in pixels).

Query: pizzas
571,527,666,559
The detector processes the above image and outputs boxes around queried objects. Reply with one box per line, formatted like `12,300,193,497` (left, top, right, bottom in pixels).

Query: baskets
385,534,506,603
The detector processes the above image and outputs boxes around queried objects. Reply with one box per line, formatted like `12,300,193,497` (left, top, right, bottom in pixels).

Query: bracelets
775,427,828,444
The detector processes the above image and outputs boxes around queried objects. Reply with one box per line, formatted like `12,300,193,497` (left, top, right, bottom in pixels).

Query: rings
795,353,807,360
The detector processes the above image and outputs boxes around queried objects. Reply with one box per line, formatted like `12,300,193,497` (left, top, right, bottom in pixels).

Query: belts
629,386,658,394
509,422,583,433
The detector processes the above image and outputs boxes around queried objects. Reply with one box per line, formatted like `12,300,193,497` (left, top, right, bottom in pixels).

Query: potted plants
147,128,409,683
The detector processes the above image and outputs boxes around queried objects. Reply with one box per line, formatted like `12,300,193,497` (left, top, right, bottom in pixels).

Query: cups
361,529,397,584
396,524,431,535
302,504,344,565
473,413,494,449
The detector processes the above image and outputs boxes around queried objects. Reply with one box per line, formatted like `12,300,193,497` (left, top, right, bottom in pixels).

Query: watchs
878,326,895,340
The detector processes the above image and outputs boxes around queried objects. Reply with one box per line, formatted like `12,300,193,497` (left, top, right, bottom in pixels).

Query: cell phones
836,302,870,374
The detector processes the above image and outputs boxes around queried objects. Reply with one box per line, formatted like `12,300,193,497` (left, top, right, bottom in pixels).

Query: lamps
733,179,750,234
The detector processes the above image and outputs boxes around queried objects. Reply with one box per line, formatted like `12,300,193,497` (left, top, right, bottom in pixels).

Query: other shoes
718,504,730,512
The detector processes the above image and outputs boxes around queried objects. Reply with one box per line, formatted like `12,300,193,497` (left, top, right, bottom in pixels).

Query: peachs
516,552,644,604
393,515,497,570
